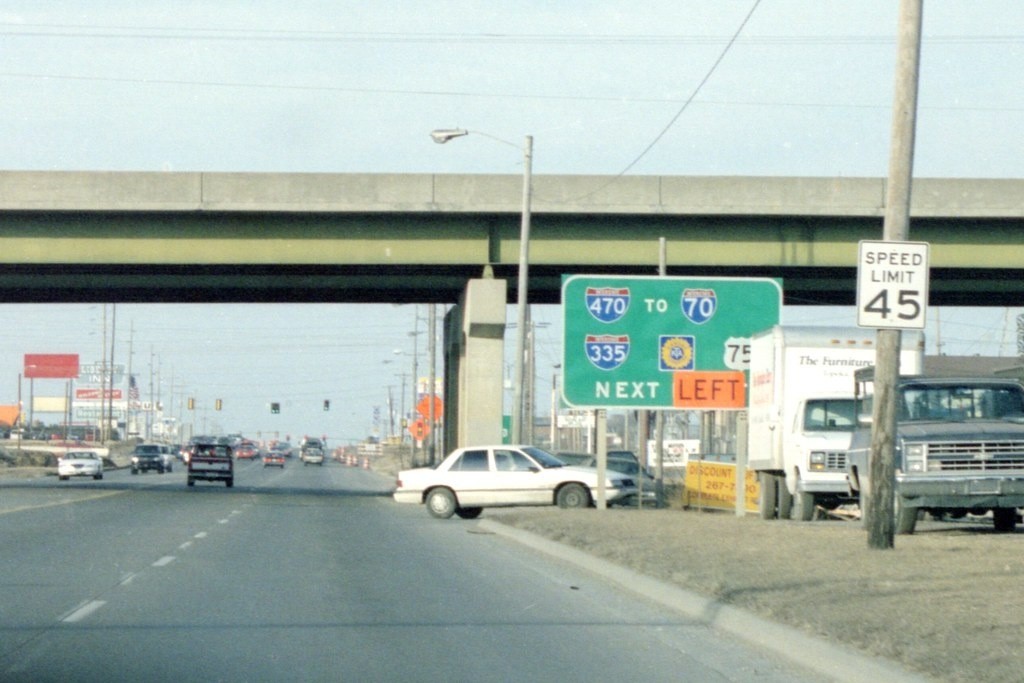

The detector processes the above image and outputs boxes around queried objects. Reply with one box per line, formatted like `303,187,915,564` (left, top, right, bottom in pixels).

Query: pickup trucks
188,443,234,488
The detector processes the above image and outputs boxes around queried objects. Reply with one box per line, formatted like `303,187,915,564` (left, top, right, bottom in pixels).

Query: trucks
299,438,322,458
747,325,926,522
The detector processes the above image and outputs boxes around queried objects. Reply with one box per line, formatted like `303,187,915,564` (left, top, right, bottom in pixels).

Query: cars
158,445,175,472
57,451,103,480
263,452,285,469
393,444,638,519
845,366,1024,535
183,445,193,465
131,445,165,474
23,431,51,441
554,452,656,508
303,448,323,466
236,447,254,461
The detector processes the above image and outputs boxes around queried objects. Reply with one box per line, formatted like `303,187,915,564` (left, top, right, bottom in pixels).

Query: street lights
394,349,427,357
432,129,534,446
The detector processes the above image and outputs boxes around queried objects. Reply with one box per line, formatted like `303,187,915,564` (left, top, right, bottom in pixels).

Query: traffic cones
347,453,358,467
362,458,370,470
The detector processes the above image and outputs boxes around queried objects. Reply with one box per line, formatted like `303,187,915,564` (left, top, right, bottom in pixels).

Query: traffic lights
271,404,279,413
189,399,193,409
216,400,220,410
324,400,329,411
